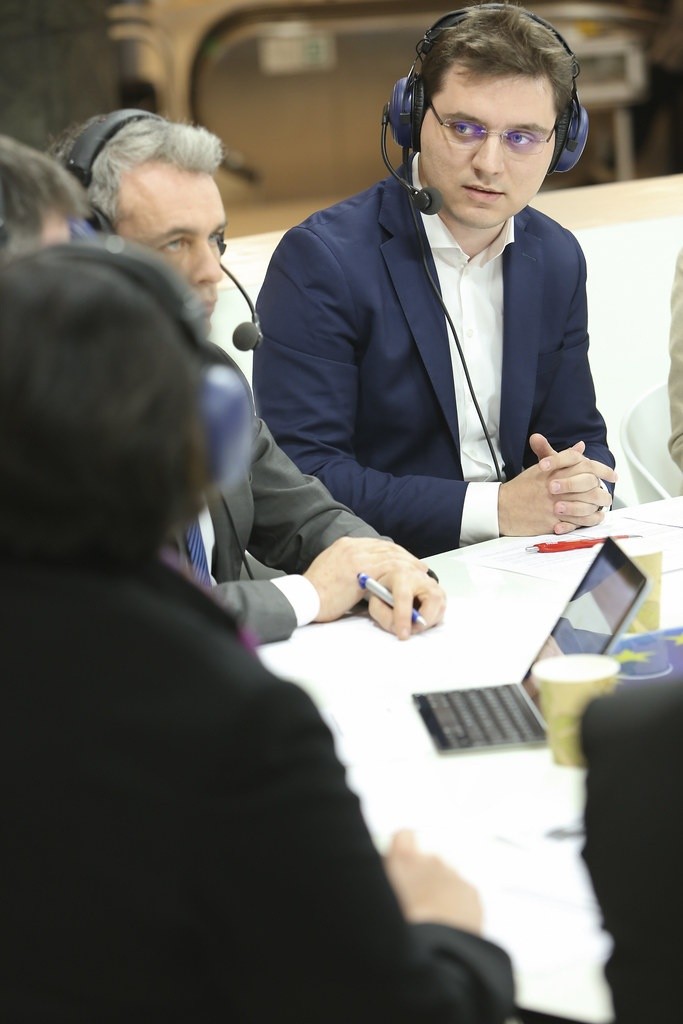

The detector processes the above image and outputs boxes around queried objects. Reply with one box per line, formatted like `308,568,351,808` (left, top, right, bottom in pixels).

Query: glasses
427,100,558,155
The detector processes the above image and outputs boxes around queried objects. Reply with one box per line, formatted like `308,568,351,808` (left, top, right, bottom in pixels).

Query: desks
246,493,683,1024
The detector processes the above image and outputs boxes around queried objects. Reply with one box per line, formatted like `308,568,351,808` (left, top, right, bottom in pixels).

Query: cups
530,652,622,766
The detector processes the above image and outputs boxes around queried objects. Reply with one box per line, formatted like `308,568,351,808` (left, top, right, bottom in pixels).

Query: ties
183,522,211,588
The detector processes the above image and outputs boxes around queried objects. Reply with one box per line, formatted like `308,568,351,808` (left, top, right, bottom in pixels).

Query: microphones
380,124,443,215
220,263,261,350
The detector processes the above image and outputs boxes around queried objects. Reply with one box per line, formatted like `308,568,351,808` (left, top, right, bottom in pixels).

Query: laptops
411,536,651,756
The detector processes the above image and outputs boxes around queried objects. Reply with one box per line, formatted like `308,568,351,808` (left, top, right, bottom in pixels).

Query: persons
0,224,527,1024
42,106,447,651
250,4,618,560
0,127,86,268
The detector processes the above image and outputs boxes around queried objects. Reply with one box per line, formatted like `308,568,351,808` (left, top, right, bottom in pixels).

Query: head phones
382,3,589,174
60,109,227,256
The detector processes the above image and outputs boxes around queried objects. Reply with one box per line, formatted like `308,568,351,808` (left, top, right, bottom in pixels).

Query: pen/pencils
357,572,427,625
526,534,642,552
